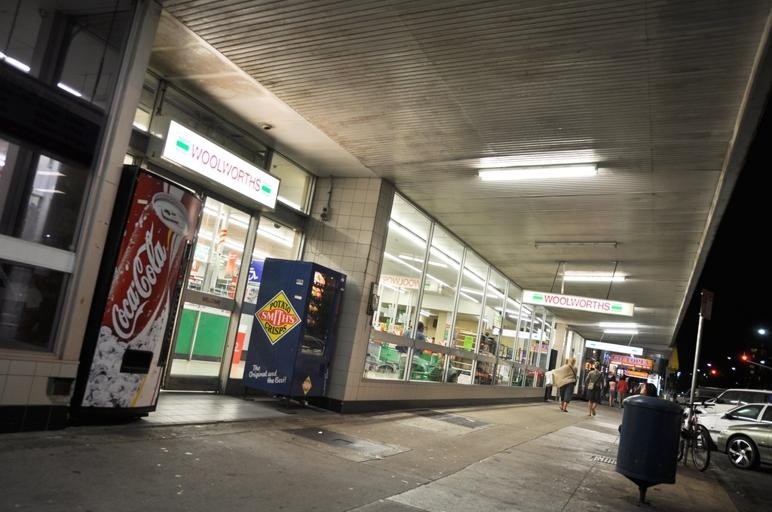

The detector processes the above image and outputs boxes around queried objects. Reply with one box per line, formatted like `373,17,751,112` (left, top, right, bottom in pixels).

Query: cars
679,387,772,470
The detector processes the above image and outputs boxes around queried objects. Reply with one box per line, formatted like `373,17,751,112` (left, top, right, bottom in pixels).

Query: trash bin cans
614,394,683,502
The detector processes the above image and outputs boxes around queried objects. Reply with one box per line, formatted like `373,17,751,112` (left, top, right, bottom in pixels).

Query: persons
395,319,427,379
438,352,458,382
558,356,658,432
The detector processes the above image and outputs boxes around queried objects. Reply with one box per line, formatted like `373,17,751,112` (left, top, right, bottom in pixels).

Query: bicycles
677,401,715,473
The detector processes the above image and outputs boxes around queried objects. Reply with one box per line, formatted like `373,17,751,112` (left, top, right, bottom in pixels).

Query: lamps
476,162,639,336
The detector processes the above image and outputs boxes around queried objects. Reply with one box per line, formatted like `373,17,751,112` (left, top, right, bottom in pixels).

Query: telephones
371,294,380,312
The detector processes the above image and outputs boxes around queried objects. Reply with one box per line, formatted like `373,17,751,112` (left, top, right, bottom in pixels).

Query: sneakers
560,405,568,412
591,408,596,416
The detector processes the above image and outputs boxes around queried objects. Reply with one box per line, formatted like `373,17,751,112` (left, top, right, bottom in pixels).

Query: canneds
101,192,190,342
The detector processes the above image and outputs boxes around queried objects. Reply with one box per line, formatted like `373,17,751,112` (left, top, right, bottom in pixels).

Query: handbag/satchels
588,382,594,390
